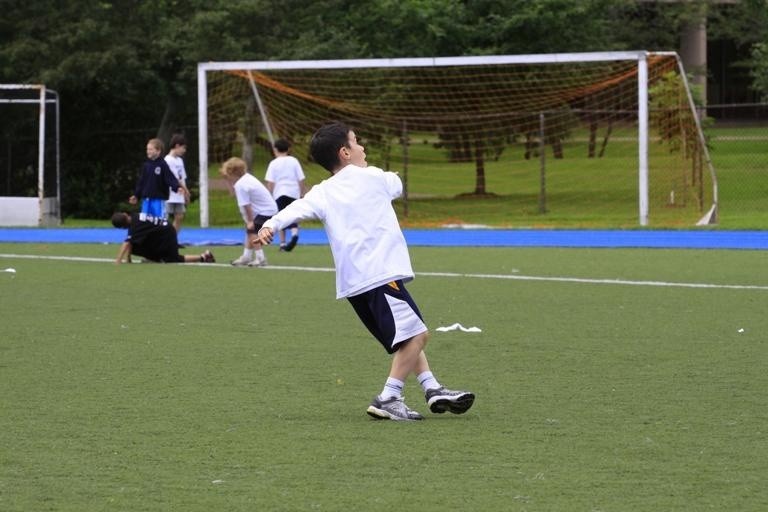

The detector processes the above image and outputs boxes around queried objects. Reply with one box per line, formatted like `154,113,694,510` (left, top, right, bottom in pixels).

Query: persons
264,140,307,252
130,138,185,263
219,156,280,267
111,211,216,264
164,132,192,249
252,121,475,421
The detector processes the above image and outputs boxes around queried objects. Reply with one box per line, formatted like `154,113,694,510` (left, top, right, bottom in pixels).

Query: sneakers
232,256,268,266
367,395,425,420
201,250,215,262
425,385,475,414
